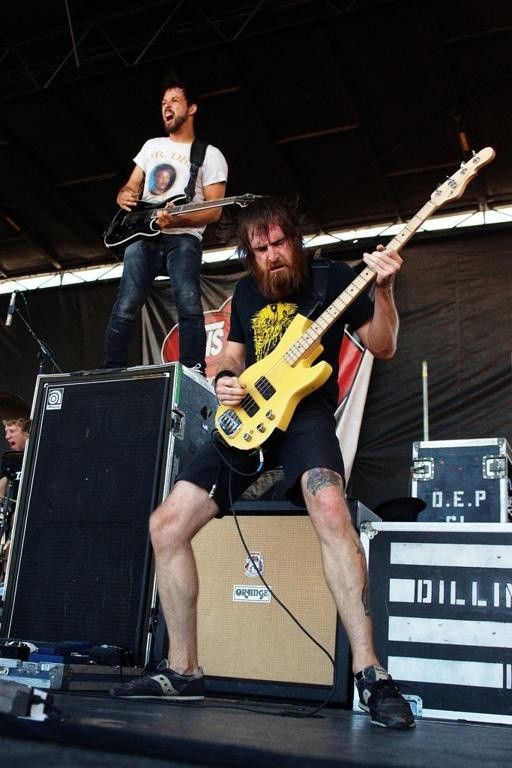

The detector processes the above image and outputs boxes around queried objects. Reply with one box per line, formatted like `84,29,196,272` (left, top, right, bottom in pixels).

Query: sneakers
111,660,206,701
356,666,417,731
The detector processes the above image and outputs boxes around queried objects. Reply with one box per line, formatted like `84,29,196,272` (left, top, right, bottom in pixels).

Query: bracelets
215,370,235,381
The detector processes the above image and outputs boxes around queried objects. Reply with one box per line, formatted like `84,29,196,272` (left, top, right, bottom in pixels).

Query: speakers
0,360,219,669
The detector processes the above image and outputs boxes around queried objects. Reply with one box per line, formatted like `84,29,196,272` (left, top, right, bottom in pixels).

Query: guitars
212,143,495,459
103,194,263,251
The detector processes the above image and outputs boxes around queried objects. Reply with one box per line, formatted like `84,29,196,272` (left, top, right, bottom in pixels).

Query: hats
372,497,426,522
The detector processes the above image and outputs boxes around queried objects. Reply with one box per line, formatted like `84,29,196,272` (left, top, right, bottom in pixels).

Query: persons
0,409,30,586
98,81,230,380
107,197,418,733
150,164,174,195
